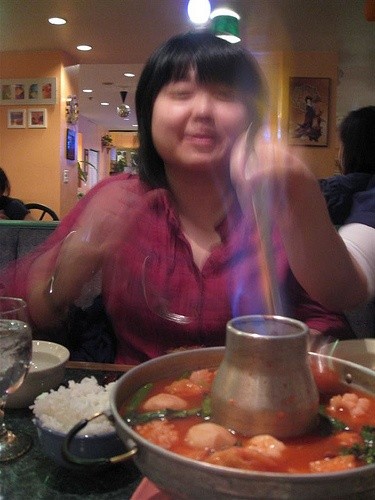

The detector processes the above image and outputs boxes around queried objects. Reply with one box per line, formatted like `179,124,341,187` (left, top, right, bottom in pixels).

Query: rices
30,375,117,435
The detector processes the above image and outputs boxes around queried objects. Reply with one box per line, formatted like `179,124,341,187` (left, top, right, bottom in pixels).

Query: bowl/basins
33,417,128,474
5,341,70,408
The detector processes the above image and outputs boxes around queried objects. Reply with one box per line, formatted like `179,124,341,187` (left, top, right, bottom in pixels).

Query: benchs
0,218,105,360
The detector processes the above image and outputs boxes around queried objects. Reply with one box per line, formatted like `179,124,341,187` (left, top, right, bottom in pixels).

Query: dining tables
1,355,180,500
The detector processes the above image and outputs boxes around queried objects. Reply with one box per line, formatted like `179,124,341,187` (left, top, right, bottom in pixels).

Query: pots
63,314,375,500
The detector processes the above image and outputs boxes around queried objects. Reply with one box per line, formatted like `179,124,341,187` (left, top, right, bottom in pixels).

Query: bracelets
47,277,54,295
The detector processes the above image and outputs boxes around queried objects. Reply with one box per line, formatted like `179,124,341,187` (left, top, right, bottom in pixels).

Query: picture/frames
0,78,57,129
288,76,332,150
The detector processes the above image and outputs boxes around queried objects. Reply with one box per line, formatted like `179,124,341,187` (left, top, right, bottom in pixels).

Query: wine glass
0,297,33,461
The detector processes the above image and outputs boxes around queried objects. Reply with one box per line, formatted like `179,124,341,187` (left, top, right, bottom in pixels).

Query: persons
0,167,37,220
0,31,375,367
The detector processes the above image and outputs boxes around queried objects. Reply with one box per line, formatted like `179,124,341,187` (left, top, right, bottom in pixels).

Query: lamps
212,8,241,44
116,90,130,116
